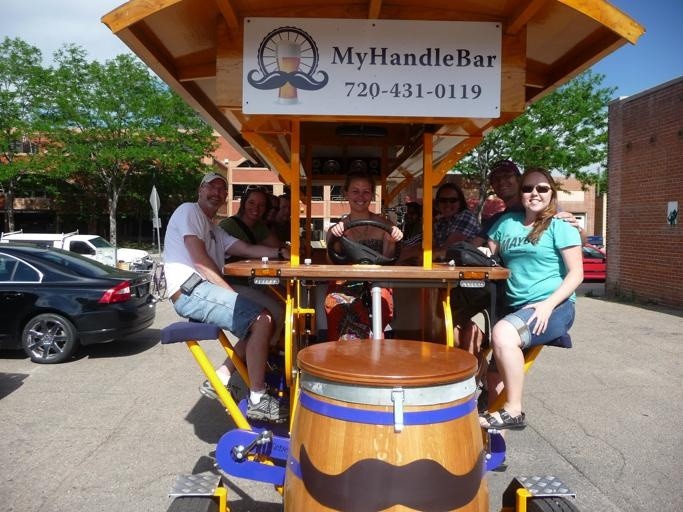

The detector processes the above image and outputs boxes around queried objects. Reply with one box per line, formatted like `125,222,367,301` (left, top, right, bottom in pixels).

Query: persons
402,161,587,355
477,166,585,432
221,189,309,368
323,175,404,340
162,172,290,423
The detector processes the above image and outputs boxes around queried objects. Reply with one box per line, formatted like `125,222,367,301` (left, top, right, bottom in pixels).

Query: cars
574,235,615,288
0,245,157,375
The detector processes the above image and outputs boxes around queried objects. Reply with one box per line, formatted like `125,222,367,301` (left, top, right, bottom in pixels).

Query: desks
221,256,512,439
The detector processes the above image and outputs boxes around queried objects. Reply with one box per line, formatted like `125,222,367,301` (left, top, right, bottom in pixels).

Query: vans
2,229,151,273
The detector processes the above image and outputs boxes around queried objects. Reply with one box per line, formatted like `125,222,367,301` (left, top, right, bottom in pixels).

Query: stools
483,331,572,470
158,321,282,494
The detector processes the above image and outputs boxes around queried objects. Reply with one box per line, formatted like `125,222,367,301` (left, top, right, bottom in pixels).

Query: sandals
482,409,526,430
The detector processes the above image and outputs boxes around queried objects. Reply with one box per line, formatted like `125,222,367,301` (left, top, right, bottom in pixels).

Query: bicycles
126,255,170,306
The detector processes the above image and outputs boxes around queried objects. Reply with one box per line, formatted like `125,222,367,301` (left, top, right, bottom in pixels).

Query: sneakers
246,393,289,423
200,381,239,405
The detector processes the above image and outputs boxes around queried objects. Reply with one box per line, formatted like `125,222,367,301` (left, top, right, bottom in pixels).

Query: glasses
440,197,459,203
521,186,551,193
245,184,268,194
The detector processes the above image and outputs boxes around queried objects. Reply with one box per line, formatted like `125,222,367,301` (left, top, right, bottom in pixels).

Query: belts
171,290,182,303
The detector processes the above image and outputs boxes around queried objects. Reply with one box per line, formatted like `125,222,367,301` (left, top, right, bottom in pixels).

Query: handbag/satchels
447,242,496,267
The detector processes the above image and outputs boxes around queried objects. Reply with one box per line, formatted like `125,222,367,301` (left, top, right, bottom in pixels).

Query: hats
200,173,227,188
488,160,517,176
